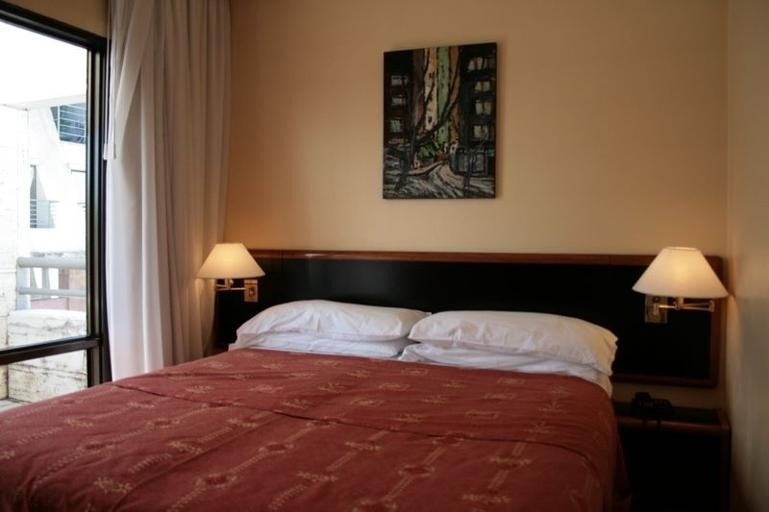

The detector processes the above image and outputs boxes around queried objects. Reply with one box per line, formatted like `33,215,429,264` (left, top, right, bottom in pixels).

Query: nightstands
615,402,734,511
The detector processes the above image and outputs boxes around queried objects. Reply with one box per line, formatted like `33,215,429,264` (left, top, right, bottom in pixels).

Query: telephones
631,392,673,419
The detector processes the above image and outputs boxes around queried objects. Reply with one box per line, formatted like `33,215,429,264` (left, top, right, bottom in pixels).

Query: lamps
631,246,730,326
195,241,265,304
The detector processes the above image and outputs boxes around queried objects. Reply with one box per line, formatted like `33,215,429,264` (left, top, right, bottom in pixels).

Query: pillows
230,299,431,341
409,309,618,376
227,336,410,359
397,344,615,398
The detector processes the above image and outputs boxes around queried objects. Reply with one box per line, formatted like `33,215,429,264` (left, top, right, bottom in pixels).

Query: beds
0,249,723,509
